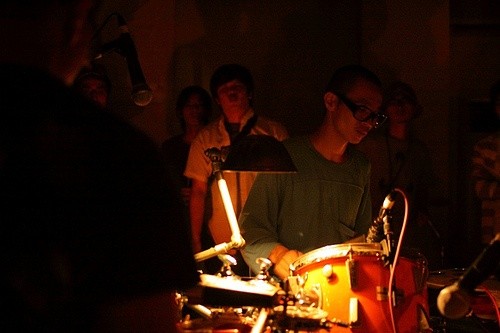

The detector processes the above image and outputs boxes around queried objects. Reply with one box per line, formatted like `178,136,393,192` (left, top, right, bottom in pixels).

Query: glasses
341,95,387,125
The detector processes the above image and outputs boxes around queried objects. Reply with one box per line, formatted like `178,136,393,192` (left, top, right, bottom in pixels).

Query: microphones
116,15,153,106
366,190,396,244
437,237,500,318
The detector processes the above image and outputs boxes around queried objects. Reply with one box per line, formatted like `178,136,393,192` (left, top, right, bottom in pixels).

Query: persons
161,84,213,206
183,62,292,286
76,73,112,108
368,81,437,257
470,78,499,281
237,62,383,283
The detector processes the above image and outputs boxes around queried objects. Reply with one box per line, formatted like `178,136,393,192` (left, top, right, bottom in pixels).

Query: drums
290,243,429,333
